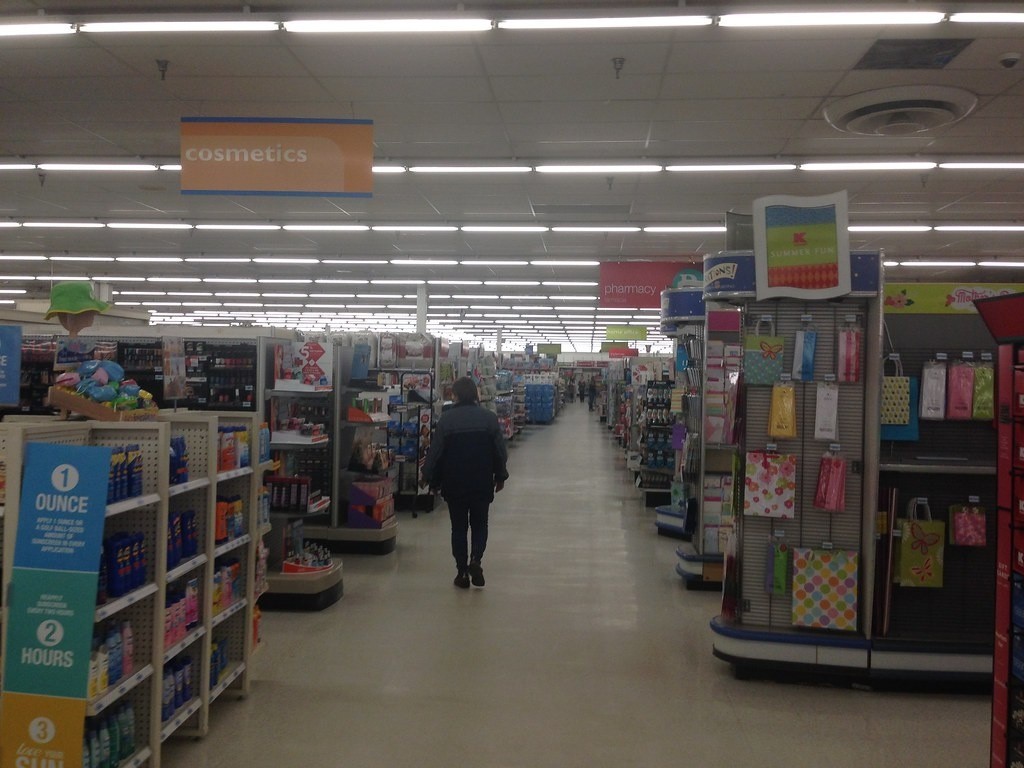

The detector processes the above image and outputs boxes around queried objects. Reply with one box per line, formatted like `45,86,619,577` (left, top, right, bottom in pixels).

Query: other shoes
469,559,486,587
454,568,470,587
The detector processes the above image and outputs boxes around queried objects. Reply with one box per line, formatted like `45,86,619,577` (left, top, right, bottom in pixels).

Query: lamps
0,154,1023,172
0,5,1024,38
0,255,1024,333
1,216,1024,236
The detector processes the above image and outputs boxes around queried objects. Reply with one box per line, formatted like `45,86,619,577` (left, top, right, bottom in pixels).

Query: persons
567,375,576,402
588,376,600,412
422,378,510,588
578,376,586,402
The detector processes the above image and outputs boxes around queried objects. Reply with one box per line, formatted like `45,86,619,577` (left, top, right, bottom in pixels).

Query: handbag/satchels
881,354,910,427
743,318,783,388
893,497,945,588
880,354,920,442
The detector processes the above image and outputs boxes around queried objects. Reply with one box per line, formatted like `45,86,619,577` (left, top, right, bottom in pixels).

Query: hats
44,281,114,320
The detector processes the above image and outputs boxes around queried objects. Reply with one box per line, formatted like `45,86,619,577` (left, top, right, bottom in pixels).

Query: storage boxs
348,476,395,528
265,475,312,511
515,384,553,423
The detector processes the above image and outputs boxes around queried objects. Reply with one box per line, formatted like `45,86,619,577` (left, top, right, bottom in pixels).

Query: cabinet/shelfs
598,390,608,423
615,424,625,444
334,345,398,555
512,382,527,433
379,335,444,513
252,334,343,593
496,390,517,439
869,282,996,677
643,424,674,508
0,411,261,768
